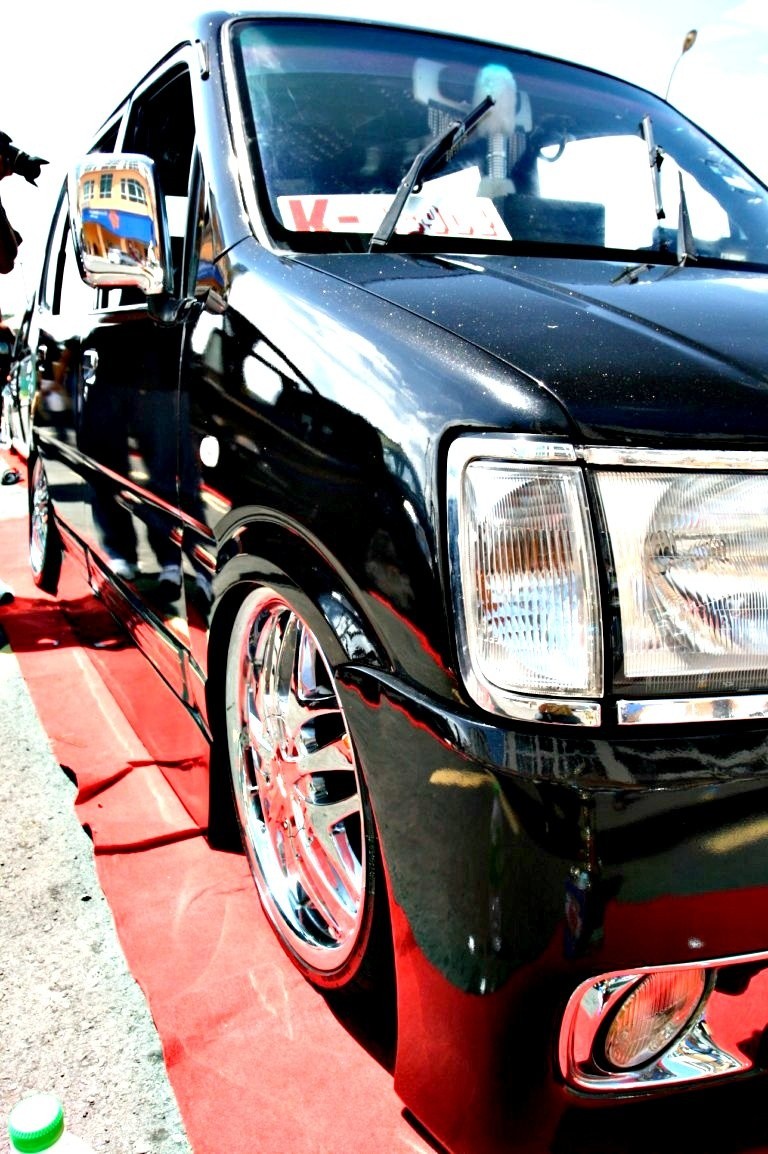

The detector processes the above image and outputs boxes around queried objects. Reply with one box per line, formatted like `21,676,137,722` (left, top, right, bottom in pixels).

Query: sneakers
106,561,139,582
158,563,184,587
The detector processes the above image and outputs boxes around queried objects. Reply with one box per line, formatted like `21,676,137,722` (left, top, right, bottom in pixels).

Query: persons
33,260,226,602
1,195,24,651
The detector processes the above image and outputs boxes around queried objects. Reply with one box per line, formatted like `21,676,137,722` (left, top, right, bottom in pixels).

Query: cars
4,8,768,1154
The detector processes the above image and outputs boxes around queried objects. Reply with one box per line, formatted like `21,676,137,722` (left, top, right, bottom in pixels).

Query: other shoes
0,578,15,605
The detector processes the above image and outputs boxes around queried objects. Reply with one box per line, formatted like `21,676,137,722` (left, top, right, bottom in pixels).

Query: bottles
10,1101,92,1154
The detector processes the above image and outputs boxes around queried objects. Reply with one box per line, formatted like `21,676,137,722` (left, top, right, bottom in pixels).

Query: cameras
0,130,50,187
